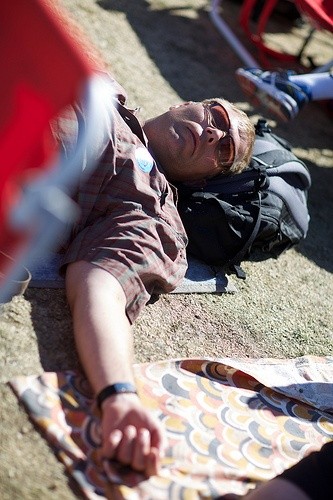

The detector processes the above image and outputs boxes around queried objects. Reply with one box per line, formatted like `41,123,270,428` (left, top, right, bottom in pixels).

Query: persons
22,70,255,476
235,67,333,123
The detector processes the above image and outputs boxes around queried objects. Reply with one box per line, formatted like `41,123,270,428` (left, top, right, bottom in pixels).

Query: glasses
207,99,237,178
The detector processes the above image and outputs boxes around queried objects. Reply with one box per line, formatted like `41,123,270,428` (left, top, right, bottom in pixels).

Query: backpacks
179,120,309,279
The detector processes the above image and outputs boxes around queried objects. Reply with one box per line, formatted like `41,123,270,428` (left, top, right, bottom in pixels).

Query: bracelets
96,383,138,408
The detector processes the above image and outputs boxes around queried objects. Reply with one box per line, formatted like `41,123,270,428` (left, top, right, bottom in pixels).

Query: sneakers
235,66,312,122
